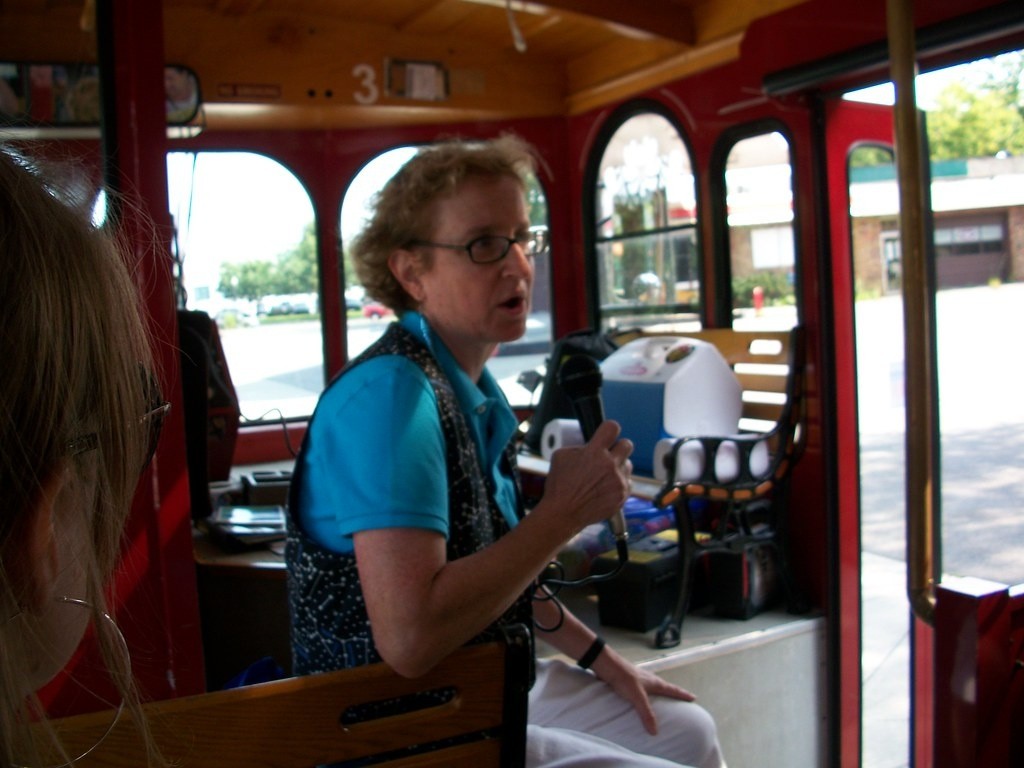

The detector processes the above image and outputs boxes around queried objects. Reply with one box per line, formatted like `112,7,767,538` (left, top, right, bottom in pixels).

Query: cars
613,276,700,304
360,300,395,318
214,309,260,328
257,286,365,314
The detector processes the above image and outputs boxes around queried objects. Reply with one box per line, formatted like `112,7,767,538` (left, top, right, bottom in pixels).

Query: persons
0,152,154,768
284,146,727,768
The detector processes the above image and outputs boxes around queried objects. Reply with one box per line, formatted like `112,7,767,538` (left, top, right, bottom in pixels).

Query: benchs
26,623,536,768
513,322,808,651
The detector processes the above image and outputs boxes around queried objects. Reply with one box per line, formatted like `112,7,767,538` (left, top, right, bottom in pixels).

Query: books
209,506,289,544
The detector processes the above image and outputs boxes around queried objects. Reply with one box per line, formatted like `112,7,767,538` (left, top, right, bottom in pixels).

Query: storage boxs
585,528,715,636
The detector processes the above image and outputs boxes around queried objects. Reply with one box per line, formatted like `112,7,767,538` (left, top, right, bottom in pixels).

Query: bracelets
578,637,605,669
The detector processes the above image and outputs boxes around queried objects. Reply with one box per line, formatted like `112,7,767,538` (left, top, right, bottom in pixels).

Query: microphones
557,353,630,566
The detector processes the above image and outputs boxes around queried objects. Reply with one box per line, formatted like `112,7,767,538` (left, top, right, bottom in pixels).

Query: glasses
406,230,548,264
52,358,173,478
